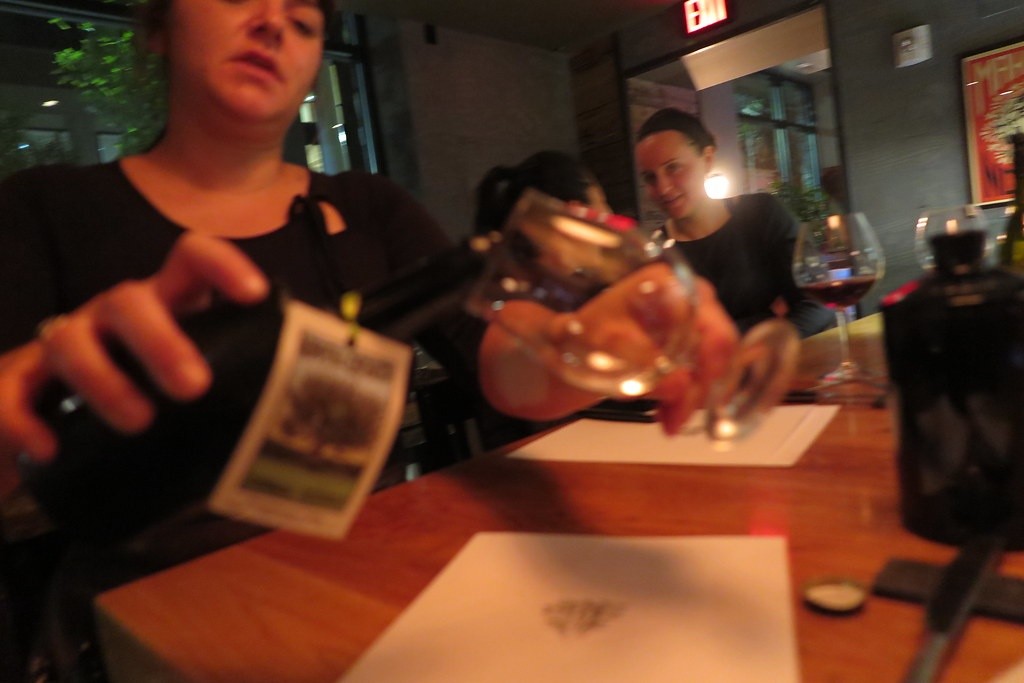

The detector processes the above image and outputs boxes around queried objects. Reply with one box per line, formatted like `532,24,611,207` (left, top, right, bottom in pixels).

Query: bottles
1001,132,1024,278
896,226,1024,550
2,228,499,584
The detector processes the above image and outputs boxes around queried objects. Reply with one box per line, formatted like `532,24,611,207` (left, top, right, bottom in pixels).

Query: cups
914,203,992,274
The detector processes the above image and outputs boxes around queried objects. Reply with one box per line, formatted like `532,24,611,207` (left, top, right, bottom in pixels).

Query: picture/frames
956,39,1024,214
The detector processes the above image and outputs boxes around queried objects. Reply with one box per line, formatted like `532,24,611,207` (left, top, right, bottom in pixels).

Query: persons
0,1,740,683
630,107,838,344
459,148,621,449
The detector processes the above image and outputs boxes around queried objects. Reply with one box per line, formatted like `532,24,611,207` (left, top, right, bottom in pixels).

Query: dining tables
89,307,1024,683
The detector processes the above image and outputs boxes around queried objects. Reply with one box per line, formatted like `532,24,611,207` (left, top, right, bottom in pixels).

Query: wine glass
793,212,887,382
464,190,801,445
